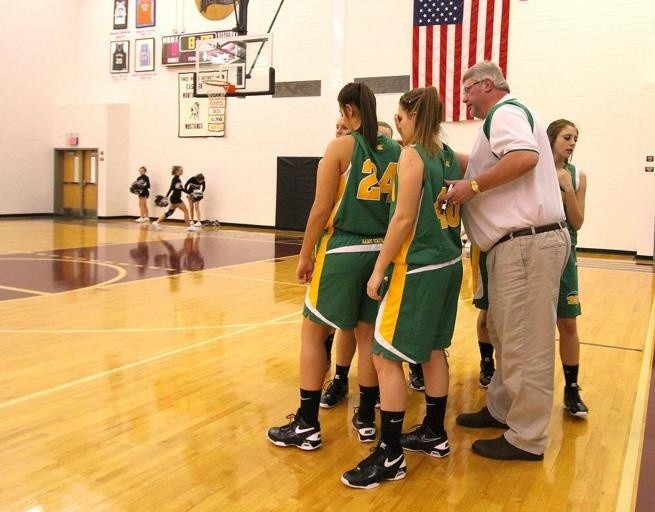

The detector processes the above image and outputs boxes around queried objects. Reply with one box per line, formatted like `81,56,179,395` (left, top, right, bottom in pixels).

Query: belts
492,221,567,246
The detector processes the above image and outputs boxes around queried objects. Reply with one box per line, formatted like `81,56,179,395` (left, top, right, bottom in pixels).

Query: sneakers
479,359,496,387
135,218,164,231
565,382,588,417
187,220,202,230
266,360,450,489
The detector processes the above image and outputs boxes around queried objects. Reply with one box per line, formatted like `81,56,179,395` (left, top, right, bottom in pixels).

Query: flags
409,1,514,122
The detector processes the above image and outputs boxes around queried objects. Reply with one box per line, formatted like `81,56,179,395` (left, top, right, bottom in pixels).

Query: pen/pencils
440,184,454,215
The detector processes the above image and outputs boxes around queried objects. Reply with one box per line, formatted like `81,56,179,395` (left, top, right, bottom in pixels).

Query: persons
183,173,206,228
133,166,151,222
130,226,204,291
433,60,573,460
335,84,470,490
151,165,196,231
311,115,496,409
541,117,590,419
265,79,403,453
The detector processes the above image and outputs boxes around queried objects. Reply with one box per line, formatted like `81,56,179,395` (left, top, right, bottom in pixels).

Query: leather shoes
456,406,511,428
472,434,543,461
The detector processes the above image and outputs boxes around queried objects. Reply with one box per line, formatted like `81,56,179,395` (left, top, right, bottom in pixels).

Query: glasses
463,82,482,95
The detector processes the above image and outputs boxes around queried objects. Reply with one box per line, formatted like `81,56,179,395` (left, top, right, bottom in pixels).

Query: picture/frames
110,1,156,74
